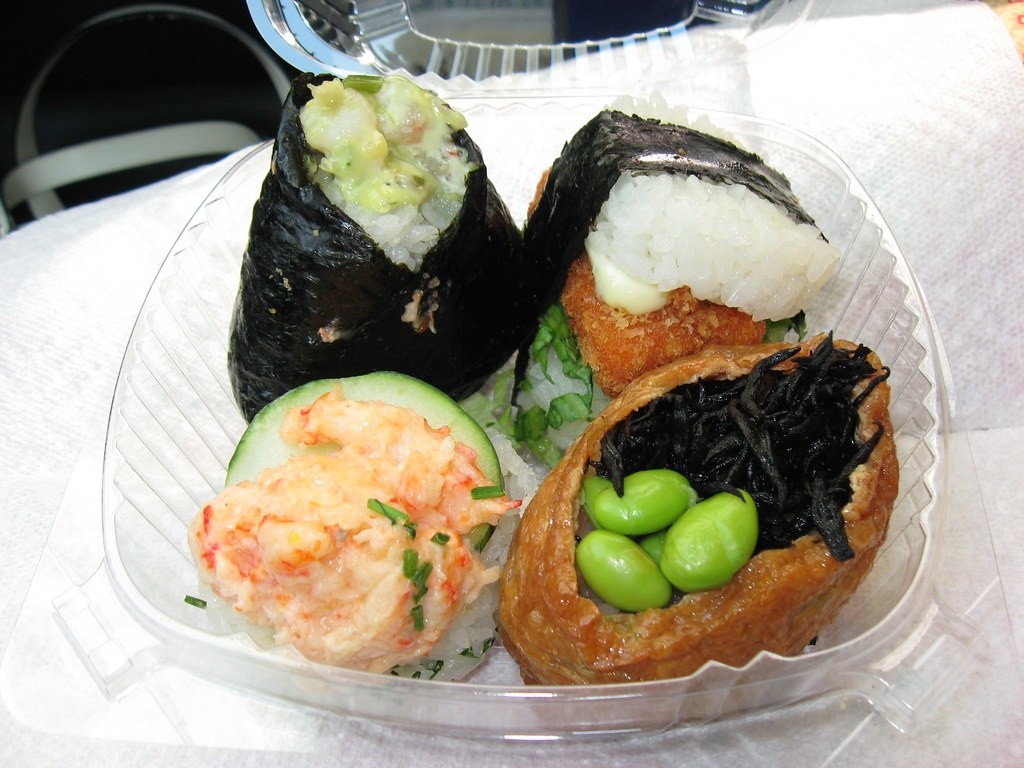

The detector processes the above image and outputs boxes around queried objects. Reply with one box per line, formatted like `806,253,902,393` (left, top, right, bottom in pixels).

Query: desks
0,0,1024,768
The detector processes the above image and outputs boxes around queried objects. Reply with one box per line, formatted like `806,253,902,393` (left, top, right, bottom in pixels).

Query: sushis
184,65,898,682
